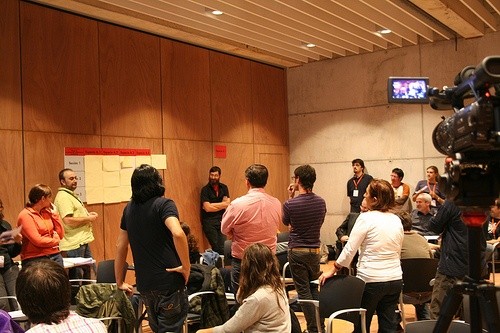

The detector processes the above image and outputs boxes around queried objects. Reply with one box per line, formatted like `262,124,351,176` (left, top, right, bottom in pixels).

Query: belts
287,247,321,254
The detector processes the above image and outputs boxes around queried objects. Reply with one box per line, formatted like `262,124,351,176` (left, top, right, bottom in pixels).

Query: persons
319,179,405,333
335,199,368,277
412,166,448,215
409,193,435,233
346,159,374,212
195,243,293,333
426,194,500,333
179,220,225,330
54,168,98,304
389,167,409,218
0,200,22,333
15,259,108,333
16,185,64,268
200,166,232,255
282,164,327,333
392,80,427,99
114,164,191,333
221,164,282,306
395,211,433,321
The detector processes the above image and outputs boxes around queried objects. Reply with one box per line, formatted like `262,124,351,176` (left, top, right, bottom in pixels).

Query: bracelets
333,262,341,271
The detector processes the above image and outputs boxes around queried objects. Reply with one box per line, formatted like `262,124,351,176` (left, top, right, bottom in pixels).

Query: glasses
291,176,296,180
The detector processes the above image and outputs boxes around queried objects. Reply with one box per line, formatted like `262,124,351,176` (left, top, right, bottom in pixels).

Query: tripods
432,201,500,333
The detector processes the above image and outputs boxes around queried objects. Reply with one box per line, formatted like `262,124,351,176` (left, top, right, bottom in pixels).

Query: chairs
0,232,490,333
